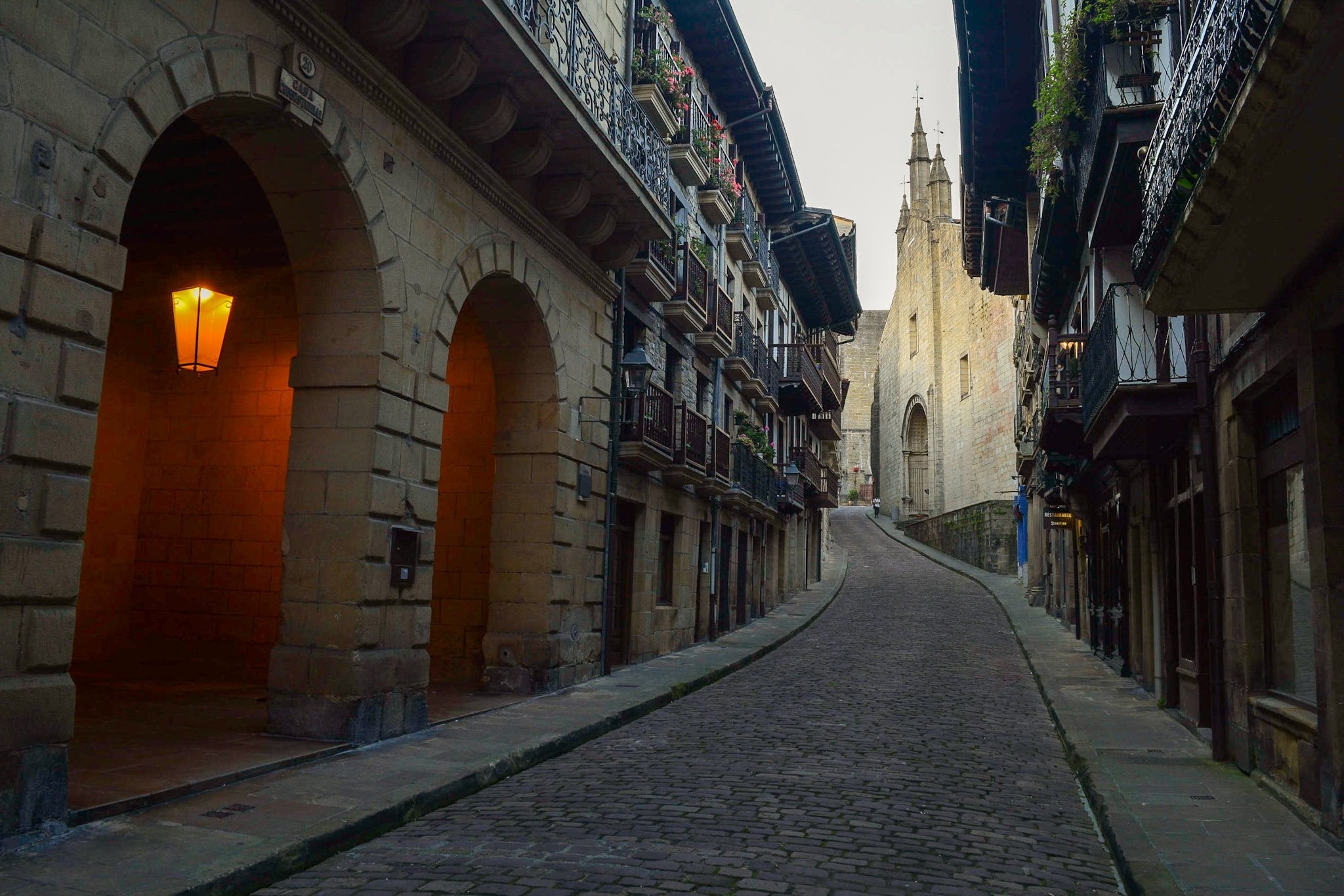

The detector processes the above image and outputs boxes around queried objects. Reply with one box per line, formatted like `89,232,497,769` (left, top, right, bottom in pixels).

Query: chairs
1114,29,1163,105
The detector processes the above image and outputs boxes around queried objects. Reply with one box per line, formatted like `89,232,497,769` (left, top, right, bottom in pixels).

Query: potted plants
847,489,859,506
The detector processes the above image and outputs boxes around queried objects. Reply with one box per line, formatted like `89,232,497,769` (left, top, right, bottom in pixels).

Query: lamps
167,259,236,380
620,338,657,404
783,461,800,487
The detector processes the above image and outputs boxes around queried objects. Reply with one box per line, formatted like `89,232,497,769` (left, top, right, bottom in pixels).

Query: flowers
632,42,695,112
733,408,748,419
636,3,674,31
692,113,743,205
736,420,776,466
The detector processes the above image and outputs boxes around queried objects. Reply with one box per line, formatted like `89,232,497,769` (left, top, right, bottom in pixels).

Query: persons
873,496,880,518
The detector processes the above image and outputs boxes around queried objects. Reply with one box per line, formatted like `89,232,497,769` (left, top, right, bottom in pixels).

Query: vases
638,16,659,33
735,418,745,425
